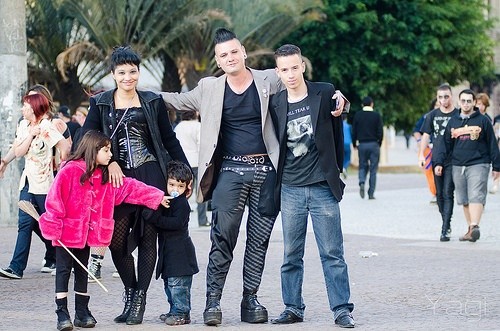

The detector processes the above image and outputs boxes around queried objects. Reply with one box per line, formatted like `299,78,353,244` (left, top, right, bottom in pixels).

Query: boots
113,288,135,321
55,296,73,331
126,290,146,324
73,293,97,328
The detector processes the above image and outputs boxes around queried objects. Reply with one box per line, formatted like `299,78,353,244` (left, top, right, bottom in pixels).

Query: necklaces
286,93,307,102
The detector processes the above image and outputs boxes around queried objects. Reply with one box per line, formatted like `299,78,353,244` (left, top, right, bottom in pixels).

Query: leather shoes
271,311,302,323
337,313,354,328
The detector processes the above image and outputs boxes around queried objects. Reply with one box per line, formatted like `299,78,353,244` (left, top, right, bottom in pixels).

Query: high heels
204,293,221,324
241,292,267,322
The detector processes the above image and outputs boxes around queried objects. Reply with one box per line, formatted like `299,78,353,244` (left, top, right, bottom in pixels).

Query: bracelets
417,137,422,143
1,158,7,165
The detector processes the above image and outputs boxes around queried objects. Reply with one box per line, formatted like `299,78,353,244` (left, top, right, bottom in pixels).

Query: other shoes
165,316,191,326
368,194,375,199
359,184,364,197
160,312,170,322
469,226,480,242
459,233,470,241
440,229,450,242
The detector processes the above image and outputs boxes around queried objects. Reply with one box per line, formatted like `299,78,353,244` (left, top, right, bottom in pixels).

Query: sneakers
0,266,21,279
51,268,56,275
41,262,55,271
87,262,101,282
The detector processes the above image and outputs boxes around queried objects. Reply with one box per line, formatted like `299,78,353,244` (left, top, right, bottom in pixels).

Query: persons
0,83,212,283
269,45,356,328
414,84,500,242
340,113,352,180
80,44,195,325
141,160,200,326
137,27,350,326
38,129,174,331
343,97,384,199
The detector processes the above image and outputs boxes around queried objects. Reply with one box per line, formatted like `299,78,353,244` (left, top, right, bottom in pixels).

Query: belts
223,154,269,164
220,166,272,175
